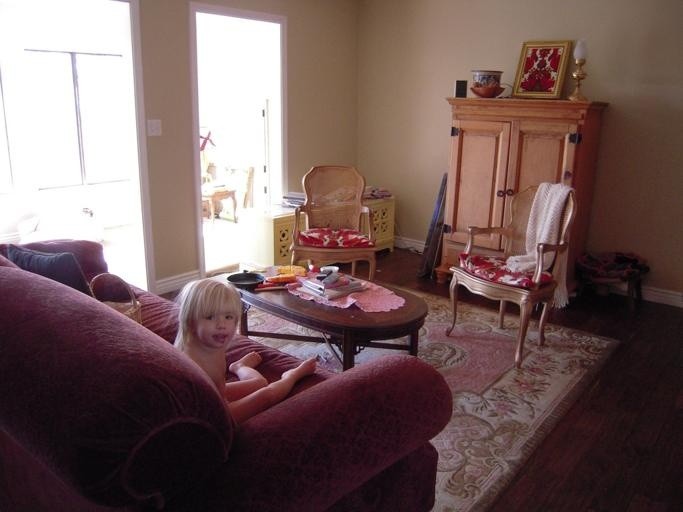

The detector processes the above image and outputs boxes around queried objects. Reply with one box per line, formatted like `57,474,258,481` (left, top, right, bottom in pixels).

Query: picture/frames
509,38,572,99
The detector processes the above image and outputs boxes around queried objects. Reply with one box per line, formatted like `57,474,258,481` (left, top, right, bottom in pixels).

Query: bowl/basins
471,71,503,87
471,88,505,97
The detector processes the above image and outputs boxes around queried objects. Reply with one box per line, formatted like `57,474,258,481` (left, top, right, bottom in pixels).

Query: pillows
297,226,374,249
3,245,95,298
457,252,556,293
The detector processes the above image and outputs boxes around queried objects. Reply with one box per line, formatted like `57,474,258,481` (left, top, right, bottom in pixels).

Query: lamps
566,38,588,101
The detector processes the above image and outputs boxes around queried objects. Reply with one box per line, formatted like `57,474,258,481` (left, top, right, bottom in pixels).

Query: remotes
322,272,339,285
316,268,331,280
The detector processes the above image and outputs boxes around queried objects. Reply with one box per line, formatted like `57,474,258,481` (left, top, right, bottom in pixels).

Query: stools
577,251,650,321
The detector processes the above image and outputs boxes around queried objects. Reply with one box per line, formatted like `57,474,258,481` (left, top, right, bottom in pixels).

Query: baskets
89,273,142,323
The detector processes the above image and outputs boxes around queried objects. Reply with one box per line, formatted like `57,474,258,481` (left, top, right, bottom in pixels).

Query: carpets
232,284,622,512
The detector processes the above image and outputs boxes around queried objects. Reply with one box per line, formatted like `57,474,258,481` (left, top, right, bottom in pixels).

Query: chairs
202,163,240,224
443,183,578,369
286,164,378,282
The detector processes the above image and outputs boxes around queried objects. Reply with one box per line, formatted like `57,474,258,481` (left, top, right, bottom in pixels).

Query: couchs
0,238,454,512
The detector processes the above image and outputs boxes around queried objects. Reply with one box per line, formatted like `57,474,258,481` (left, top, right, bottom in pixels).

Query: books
281,191,307,209
303,276,362,299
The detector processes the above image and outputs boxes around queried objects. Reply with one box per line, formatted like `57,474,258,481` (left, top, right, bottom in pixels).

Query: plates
226,273,263,289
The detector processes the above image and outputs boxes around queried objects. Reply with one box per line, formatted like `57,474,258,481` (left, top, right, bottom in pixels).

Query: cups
320,265,340,283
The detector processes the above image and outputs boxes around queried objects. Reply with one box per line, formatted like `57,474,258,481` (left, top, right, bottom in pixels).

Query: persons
168,278,317,424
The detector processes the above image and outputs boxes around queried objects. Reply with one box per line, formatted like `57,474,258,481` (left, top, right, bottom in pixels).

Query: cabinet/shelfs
260,194,395,270
425,97,610,312
